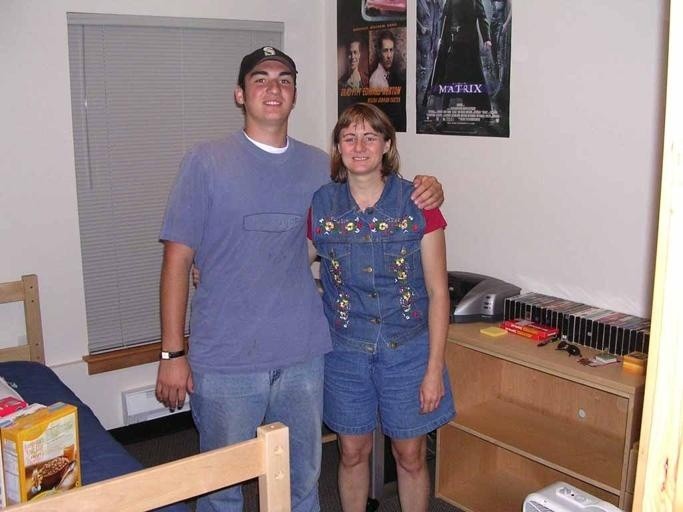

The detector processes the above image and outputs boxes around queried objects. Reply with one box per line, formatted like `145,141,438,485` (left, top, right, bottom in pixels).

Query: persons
156,45,445,512
339,29,396,105
191,101,456,512
421,0,501,135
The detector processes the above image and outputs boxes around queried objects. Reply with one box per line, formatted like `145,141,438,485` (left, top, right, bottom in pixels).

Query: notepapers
479,326,508,337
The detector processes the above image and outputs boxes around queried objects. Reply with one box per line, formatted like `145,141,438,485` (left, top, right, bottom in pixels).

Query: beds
0,274,291,512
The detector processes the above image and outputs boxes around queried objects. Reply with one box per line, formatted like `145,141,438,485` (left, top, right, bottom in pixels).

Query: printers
447,271,522,323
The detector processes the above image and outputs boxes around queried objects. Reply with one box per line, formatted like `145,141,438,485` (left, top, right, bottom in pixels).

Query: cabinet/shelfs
434,322,645,512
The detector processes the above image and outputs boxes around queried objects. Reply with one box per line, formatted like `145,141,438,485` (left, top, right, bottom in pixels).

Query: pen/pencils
537,337,558,347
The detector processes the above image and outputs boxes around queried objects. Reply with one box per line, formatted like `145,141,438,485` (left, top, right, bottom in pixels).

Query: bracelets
159,350,187,360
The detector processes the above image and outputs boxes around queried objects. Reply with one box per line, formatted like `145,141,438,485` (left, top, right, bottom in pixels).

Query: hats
239,46,298,85
